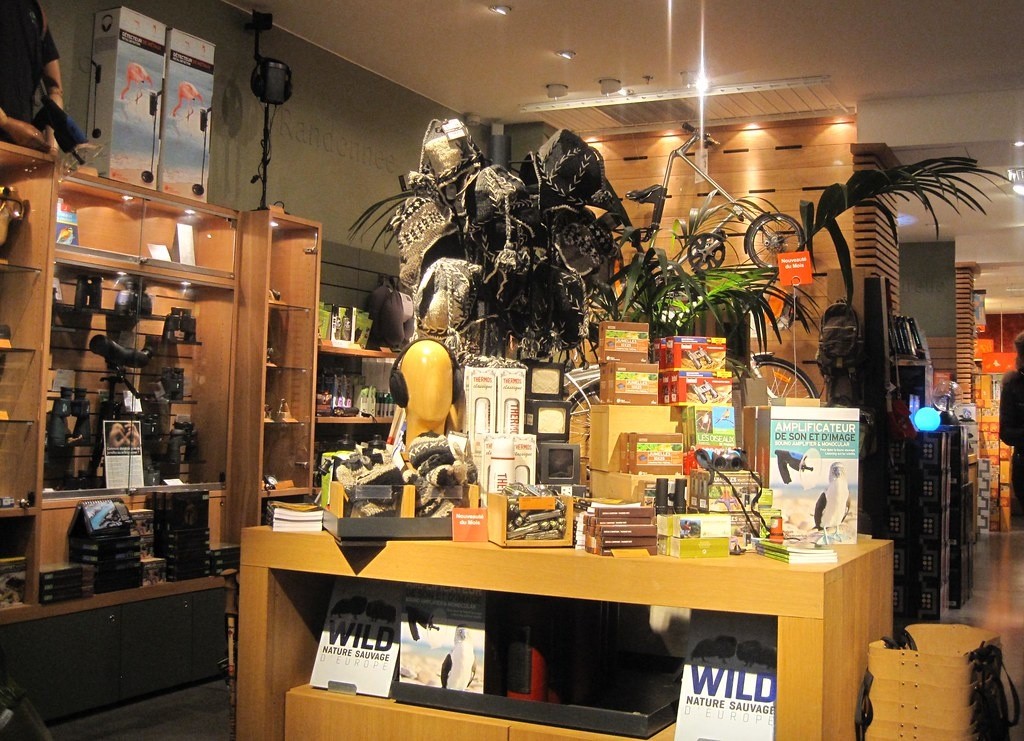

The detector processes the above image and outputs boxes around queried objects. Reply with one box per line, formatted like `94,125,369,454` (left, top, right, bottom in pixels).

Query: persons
998,332,1024,501
0,0,61,151
109,423,139,447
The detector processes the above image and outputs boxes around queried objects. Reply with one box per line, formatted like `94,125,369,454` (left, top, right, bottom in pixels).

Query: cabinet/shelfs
0,142,59,625
0,586,227,722
314,344,400,495
231,211,323,497
28,141,241,623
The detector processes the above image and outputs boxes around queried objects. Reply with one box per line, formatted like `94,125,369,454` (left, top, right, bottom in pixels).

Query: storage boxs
40,488,240,602
464,364,580,488
487,493,573,549
398,583,510,697
84,6,217,203
589,322,860,556
883,425,974,618
674,610,778,741
973,375,1012,532
855,623,1020,741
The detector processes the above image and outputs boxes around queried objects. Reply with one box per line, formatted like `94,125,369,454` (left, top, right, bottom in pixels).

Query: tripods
81,375,142,489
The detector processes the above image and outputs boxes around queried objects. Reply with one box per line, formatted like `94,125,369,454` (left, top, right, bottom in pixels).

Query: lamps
982,302,1018,373
914,407,941,434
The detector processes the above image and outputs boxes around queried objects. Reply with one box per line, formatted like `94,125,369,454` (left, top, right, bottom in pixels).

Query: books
265,501,324,533
571,495,840,565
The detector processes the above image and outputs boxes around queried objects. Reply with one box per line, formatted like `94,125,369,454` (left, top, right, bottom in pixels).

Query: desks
236,526,892,741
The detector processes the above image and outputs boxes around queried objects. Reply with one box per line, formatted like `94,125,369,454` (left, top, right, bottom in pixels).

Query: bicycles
625,121,820,398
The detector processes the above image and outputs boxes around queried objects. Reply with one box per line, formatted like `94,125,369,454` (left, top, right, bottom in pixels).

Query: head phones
389,338,463,408
